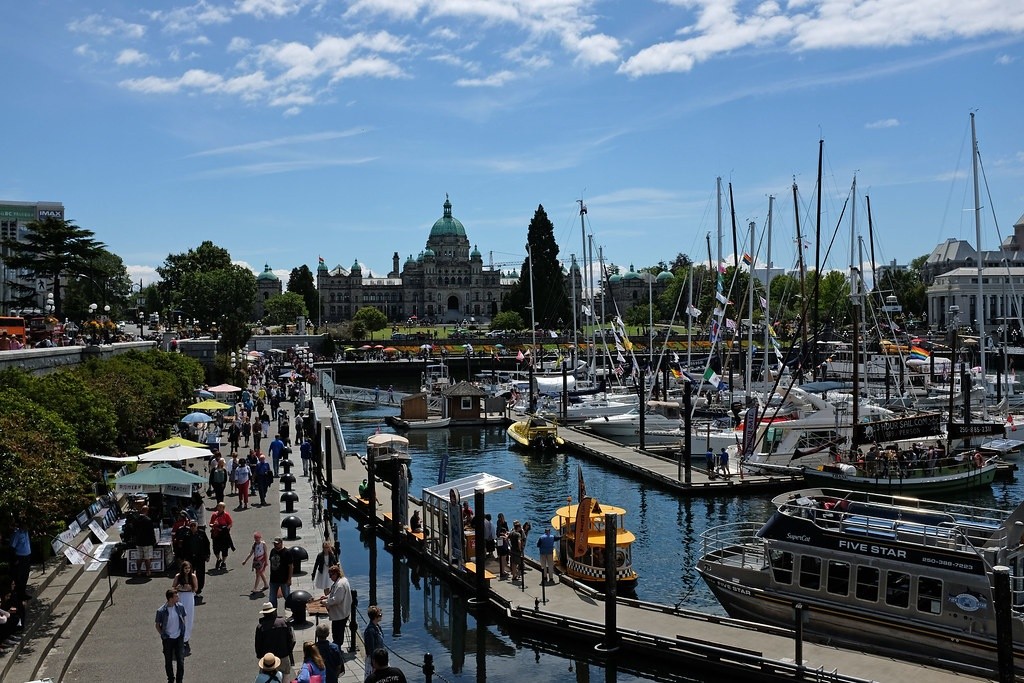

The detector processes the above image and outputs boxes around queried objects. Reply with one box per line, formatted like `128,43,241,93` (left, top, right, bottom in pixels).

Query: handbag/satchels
206,487,212,497
290,663,322,683
253,561,262,569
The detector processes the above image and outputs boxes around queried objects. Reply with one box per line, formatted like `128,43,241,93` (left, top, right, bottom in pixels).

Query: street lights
79,274,105,310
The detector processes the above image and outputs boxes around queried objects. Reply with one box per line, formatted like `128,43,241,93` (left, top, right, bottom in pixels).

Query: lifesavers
974,452,984,468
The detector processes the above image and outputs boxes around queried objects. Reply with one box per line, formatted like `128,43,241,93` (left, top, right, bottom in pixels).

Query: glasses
190,524,197,527
377,614,382,617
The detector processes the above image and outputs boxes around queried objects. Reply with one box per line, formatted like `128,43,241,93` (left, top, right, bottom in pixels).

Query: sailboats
424,109,1024,498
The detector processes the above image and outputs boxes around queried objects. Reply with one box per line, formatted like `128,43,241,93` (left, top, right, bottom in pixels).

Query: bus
0,316,26,344
30,317,66,343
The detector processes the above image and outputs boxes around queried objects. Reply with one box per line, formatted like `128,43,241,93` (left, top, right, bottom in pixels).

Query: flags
1003,415,1018,432
574,496,591,557
666,253,931,456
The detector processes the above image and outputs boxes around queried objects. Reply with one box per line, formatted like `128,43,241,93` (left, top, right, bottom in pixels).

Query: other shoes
244,504,247,510
500,571,510,580
216,558,222,569
234,505,242,512
219,563,226,568
260,586,269,590
549,580,555,584
0,628,22,652
19,594,32,600
183,641,190,655
541,580,547,585
251,587,259,592
512,576,520,581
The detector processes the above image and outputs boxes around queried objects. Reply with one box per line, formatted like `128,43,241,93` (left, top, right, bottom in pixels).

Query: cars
119,320,142,328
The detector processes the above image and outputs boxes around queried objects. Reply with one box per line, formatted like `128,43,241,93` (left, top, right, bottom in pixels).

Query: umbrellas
245,347,263,369
878,340,891,345
963,338,978,344
278,372,303,378
112,381,242,496
343,343,504,351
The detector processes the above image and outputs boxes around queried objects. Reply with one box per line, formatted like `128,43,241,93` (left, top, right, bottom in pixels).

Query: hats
217,502,226,507
413,510,420,516
259,602,277,613
189,519,197,525
272,537,283,544
258,652,282,671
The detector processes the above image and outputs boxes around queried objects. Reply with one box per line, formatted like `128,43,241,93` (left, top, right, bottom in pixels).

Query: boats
551,460,639,585
694,486,1024,683
391,413,451,428
367,426,412,474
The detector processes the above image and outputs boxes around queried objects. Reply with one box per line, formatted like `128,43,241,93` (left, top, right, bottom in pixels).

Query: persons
12,527,33,599
705,447,731,480
828,439,984,480
390,319,446,338
820,501,835,510
253,346,679,371
132,506,156,577
155,368,405,683
439,499,566,588
0,326,203,362
410,510,429,536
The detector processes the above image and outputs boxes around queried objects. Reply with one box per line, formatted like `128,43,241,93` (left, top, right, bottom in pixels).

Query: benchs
463,562,496,588
405,527,424,541
382,512,392,525
354,496,369,507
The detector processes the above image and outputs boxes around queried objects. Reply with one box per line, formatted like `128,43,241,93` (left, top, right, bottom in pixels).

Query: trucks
149,319,159,330
